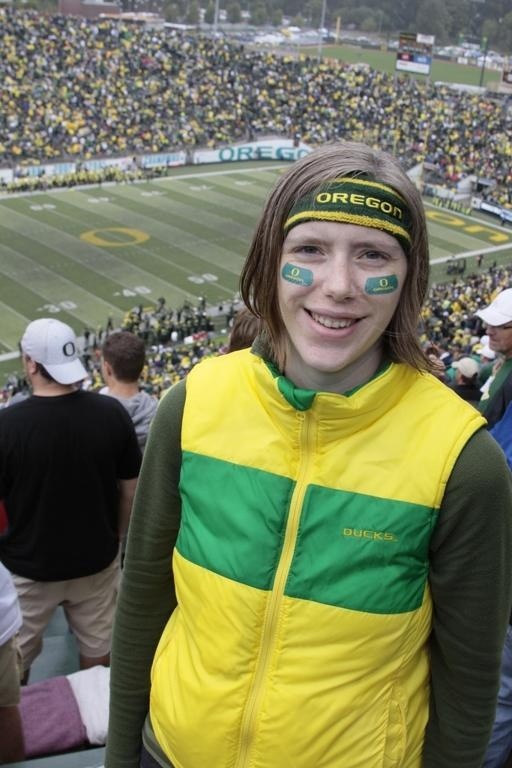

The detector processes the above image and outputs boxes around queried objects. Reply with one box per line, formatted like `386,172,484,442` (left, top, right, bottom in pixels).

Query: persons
0,4,512,210
409,252,512,465
103,143,512,768
0,294,242,763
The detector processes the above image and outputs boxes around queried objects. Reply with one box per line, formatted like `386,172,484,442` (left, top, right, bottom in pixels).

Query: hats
476,343,495,359
473,288,512,327
451,357,478,378
21,317,90,386
